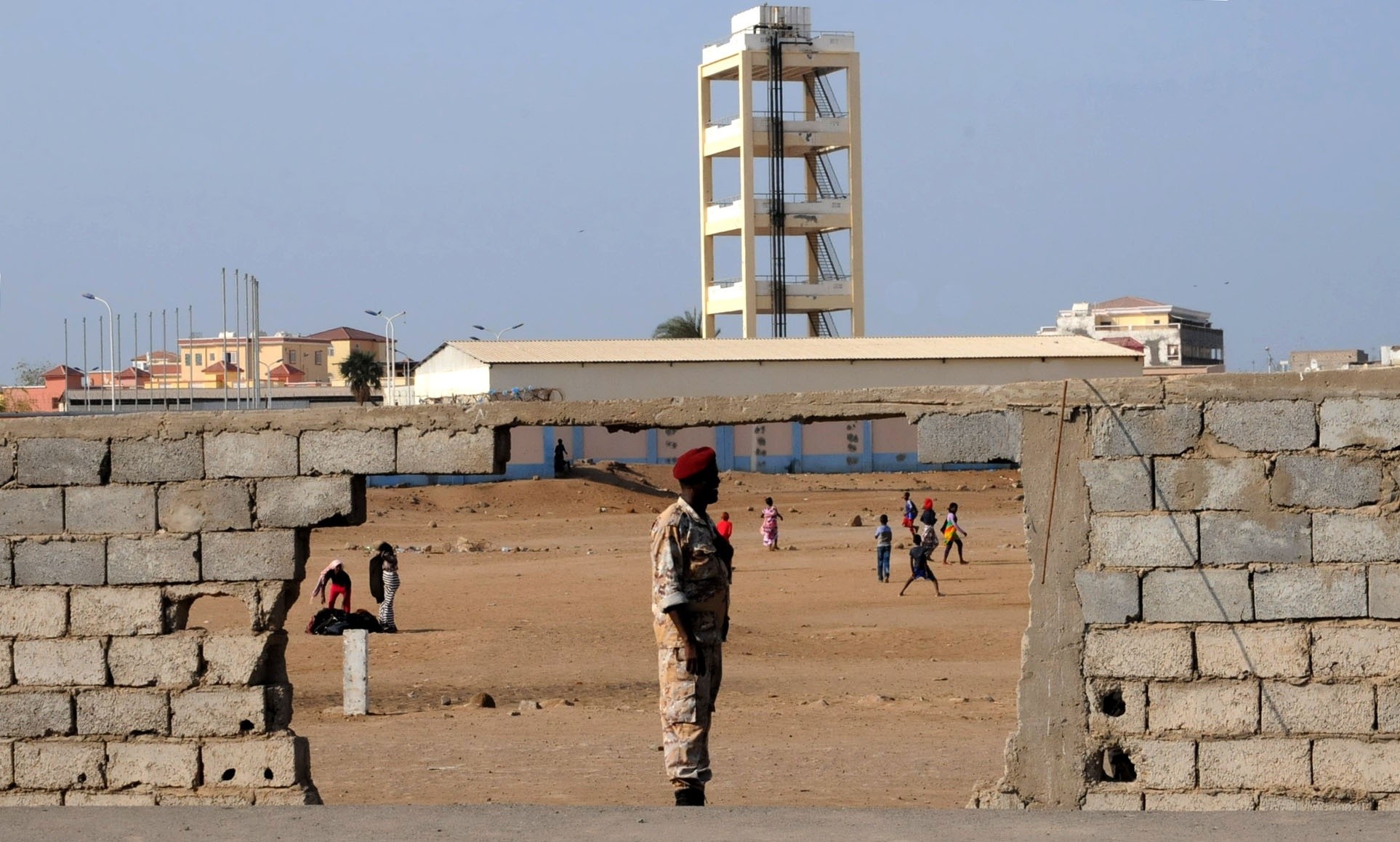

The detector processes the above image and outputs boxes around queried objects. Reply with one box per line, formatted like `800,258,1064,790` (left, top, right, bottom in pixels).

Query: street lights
473,322,524,340
364,309,396,407
81,292,116,412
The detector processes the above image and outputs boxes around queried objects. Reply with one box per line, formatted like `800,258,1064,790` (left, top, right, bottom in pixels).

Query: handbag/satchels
940,519,947,533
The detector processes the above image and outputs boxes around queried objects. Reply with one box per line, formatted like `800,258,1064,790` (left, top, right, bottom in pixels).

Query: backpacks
911,501,919,519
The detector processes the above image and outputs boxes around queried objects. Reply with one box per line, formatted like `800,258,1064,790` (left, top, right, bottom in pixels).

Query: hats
673,446,716,483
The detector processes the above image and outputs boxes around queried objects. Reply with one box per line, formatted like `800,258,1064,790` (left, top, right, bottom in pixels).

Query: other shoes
675,789,705,806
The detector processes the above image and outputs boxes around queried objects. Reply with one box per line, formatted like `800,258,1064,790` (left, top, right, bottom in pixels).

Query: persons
760,497,783,551
920,498,939,561
901,492,919,535
555,438,568,472
320,560,351,612
370,541,401,633
875,514,892,583
716,512,733,543
899,534,945,596
940,502,968,565
650,446,733,807
